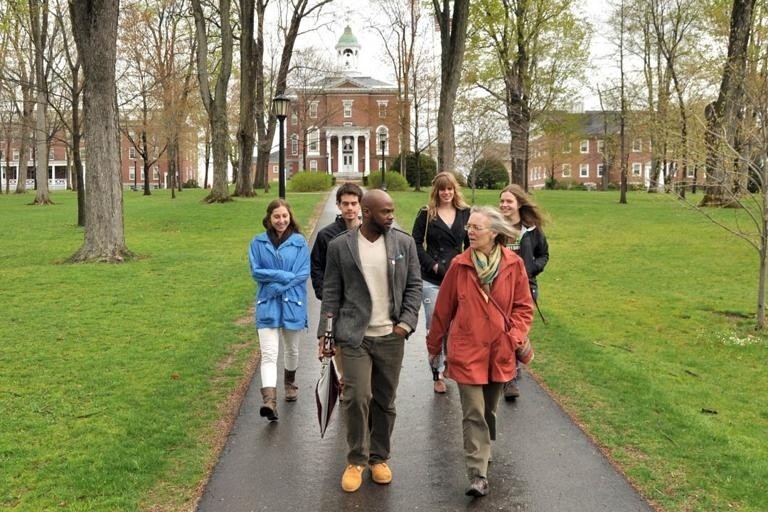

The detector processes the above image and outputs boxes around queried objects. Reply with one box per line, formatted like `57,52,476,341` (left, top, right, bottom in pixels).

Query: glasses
464,224,485,231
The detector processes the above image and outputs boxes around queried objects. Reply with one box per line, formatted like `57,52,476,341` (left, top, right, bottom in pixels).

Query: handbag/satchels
516,334,535,367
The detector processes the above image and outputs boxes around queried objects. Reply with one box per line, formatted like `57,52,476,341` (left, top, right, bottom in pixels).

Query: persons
496,182,552,400
310,182,363,302
316,188,425,493
426,202,537,499
410,170,474,395
247,197,312,422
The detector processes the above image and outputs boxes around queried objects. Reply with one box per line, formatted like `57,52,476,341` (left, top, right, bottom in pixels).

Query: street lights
272,90,291,199
377,129,390,191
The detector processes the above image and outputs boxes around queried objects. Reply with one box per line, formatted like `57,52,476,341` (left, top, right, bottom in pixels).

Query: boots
283,367,300,402
259,386,279,420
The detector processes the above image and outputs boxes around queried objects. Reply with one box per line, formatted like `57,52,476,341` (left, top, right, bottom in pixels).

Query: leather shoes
340,463,366,493
464,477,490,497
368,460,392,484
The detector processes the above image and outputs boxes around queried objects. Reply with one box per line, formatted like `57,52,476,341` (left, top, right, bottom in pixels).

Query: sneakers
502,380,520,399
516,367,523,379
433,380,447,393
